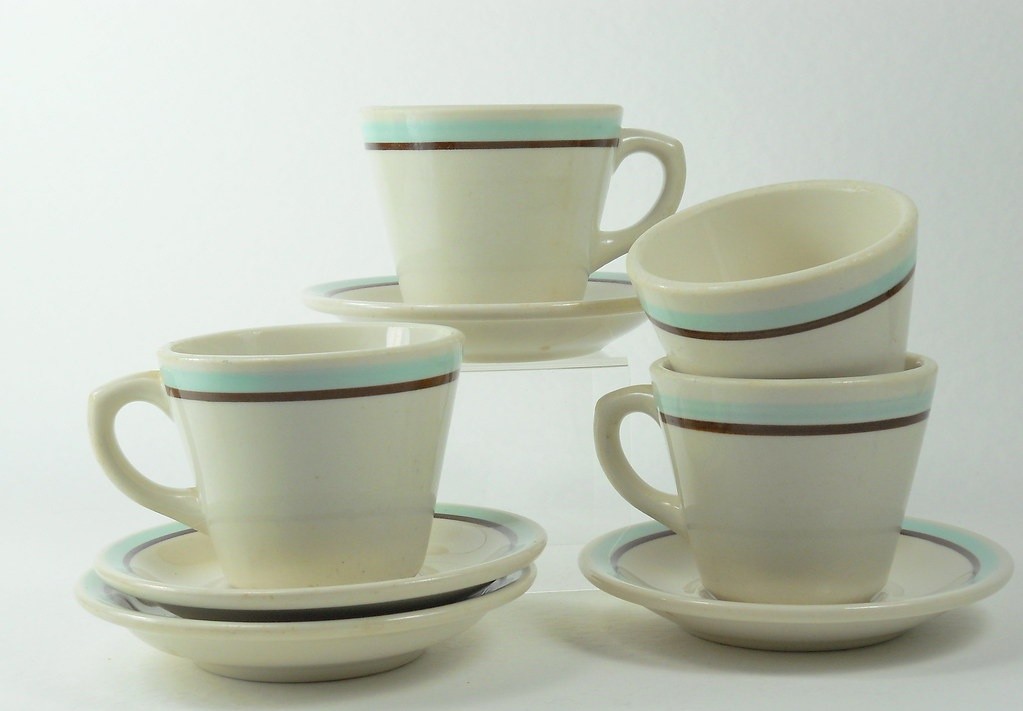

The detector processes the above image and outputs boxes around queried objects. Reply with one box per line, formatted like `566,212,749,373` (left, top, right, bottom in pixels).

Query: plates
580,515,1014,652
304,273,654,364
72,502,545,685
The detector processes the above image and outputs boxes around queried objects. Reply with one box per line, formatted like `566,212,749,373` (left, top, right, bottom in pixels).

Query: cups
88,322,463,587
594,351,937,603
364,105,687,303
625,180,918,376
